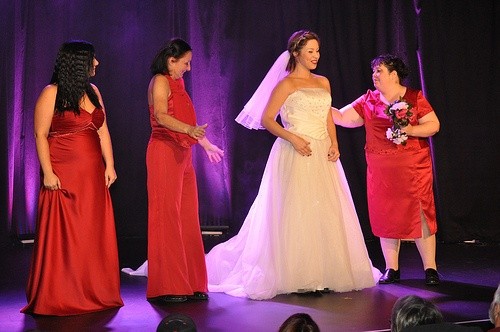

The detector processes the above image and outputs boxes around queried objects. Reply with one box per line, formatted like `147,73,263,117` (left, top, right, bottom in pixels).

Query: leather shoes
378,268,400,284
424,268,439,284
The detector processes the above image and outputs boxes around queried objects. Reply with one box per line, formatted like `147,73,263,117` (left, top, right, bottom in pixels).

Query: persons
157,286,500,332
20,41,124,316
331,55,443,285
146,38,224,304
260,30,341,300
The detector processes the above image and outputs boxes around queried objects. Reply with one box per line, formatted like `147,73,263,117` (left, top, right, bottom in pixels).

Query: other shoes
161,293,208,302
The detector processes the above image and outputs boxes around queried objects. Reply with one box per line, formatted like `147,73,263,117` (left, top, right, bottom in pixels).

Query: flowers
383,96,414,147
